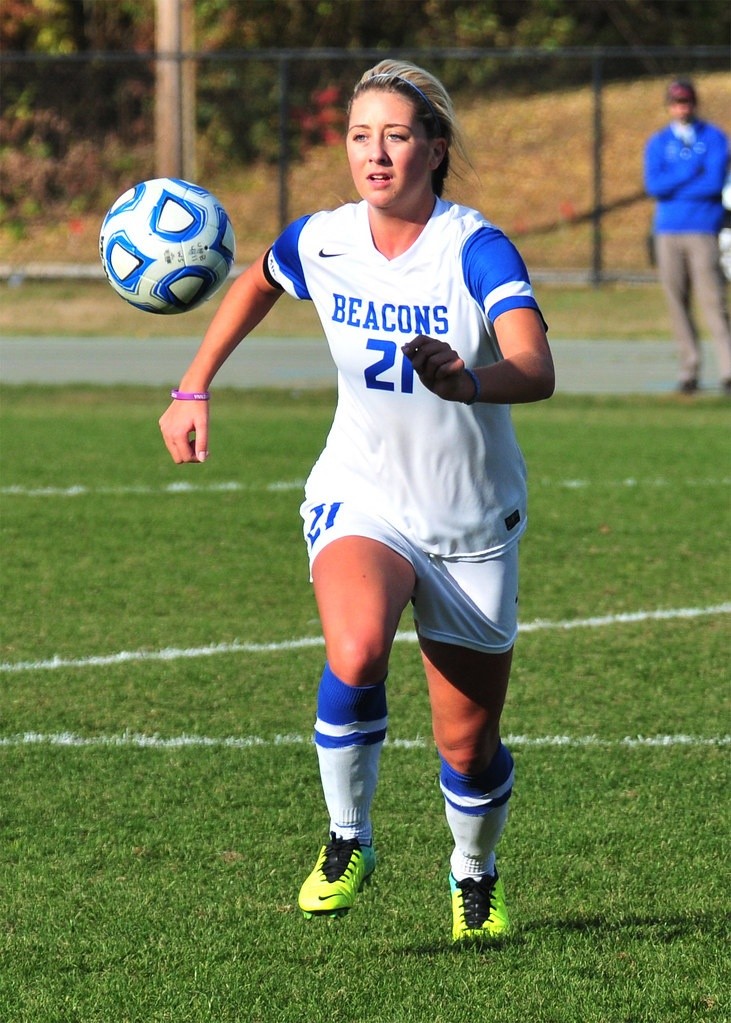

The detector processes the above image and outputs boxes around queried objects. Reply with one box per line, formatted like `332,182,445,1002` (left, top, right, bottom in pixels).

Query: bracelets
464,369,480,405
170,389,210,400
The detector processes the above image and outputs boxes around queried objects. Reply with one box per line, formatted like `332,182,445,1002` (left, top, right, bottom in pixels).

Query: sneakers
448,869,512,949
297,839,375,919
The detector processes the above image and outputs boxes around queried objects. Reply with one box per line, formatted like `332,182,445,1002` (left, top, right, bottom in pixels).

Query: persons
644,79,731,395
155,60,557,941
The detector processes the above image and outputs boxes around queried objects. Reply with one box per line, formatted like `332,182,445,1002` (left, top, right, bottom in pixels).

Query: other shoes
721,379,731,393
679,380,696,395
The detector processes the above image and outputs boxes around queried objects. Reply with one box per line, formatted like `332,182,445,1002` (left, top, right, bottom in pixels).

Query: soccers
95,177,236,316
